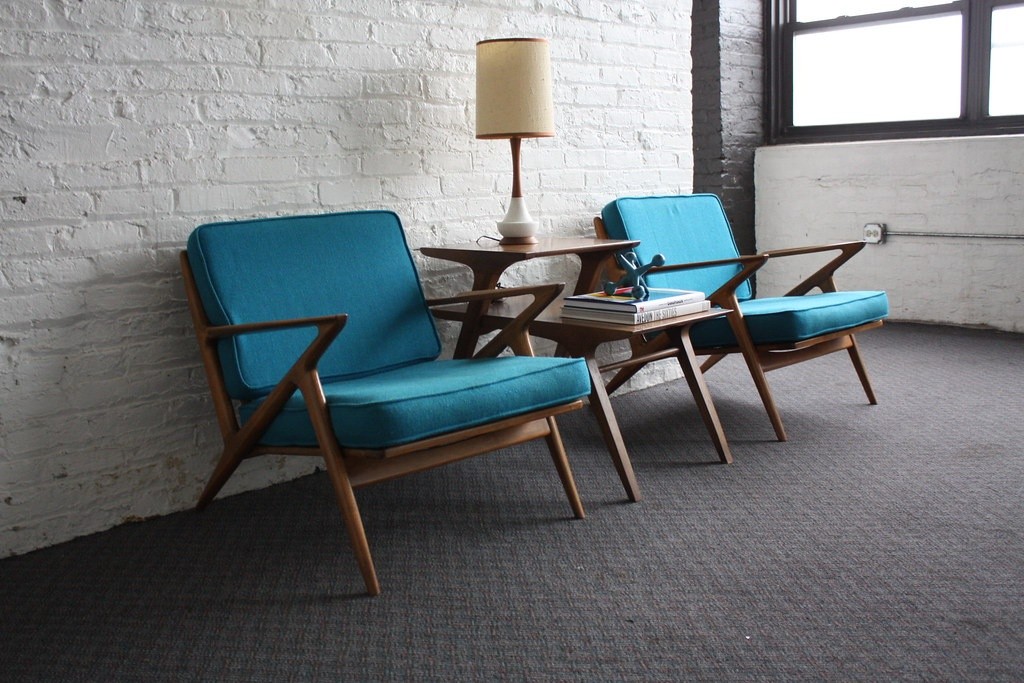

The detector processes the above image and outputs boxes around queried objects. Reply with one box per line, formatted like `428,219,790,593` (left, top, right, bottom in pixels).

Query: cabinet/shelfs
418,238,735,502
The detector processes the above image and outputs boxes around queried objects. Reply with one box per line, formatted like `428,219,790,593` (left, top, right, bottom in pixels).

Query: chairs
178,210,593,597
593,195,890,442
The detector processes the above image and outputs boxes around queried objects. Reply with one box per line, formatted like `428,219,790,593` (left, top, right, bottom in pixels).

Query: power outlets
863,223,886,244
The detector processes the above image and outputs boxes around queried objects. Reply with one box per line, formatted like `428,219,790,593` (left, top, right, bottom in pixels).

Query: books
558,284,711,326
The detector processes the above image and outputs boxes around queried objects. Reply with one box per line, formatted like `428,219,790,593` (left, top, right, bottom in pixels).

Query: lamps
475,38,555,244
